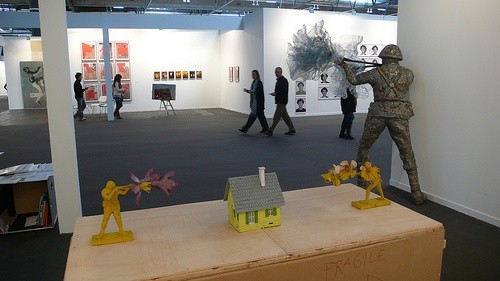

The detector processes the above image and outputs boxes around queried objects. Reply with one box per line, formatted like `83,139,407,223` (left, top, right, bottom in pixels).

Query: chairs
73,96,116,117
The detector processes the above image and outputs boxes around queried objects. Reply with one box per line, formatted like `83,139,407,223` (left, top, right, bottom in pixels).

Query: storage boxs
63,182,446,281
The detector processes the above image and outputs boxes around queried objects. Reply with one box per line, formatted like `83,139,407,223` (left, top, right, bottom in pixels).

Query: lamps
305,6,314,13
343,9,356,16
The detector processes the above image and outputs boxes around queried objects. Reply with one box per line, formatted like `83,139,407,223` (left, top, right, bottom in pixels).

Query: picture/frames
80,40,132,103
295,44,386,115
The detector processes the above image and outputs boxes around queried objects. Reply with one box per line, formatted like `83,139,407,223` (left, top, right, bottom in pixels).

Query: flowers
127,168,178,206
320,158,382,187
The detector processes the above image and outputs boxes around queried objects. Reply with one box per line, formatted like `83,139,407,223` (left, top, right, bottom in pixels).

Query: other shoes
260,128,268,133
284,129,296,135
114,111,123,119
78,118,86,120
339,133,355,139
238,127,247,133
263,131,273,136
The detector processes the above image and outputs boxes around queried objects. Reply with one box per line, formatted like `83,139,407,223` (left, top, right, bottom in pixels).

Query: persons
360,45,367,55
73,72,89,121
320,73,330,83
336,44,427,205
263,67,296,135
96,180,130,239
238,70,269,135
296,82,306,95
339,87,357,140
112,74,126,119
371,45,379,55
320,87,329,98
296,99,307,112
358,162,385,204
